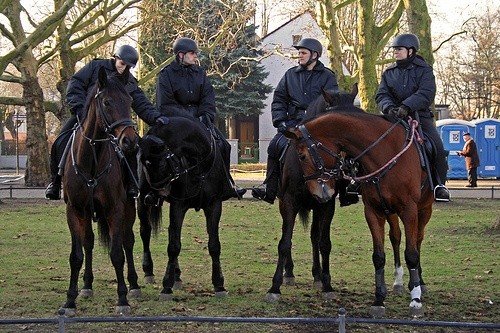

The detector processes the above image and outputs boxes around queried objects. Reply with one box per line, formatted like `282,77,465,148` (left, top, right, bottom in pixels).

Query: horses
266,82,436,319
137,106,227,303
63,64,144,317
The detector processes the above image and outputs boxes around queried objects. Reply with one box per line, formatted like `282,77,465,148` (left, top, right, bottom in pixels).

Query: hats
463,133,470,136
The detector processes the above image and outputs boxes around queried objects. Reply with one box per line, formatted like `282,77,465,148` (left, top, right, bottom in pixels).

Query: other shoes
45,180,61,200
339,193,359,204
433,186,450,202
232,188,246,198
252,188,276,205
144,191,164,206
464,184,477,187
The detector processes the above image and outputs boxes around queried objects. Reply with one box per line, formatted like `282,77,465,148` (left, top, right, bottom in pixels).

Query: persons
347,34,450,201
143,37,245,206
252,37,359,206
46,46,168,200
457,133,480,187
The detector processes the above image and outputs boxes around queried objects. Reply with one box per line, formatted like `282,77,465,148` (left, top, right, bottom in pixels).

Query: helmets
294,38,322,57
392,34,420,51
115,45,139,68
173,38,200,56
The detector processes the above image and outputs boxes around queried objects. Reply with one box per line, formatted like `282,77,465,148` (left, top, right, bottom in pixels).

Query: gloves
387,105,410,118
155,116,170,127
278,121,287,133
74,108,84,120
